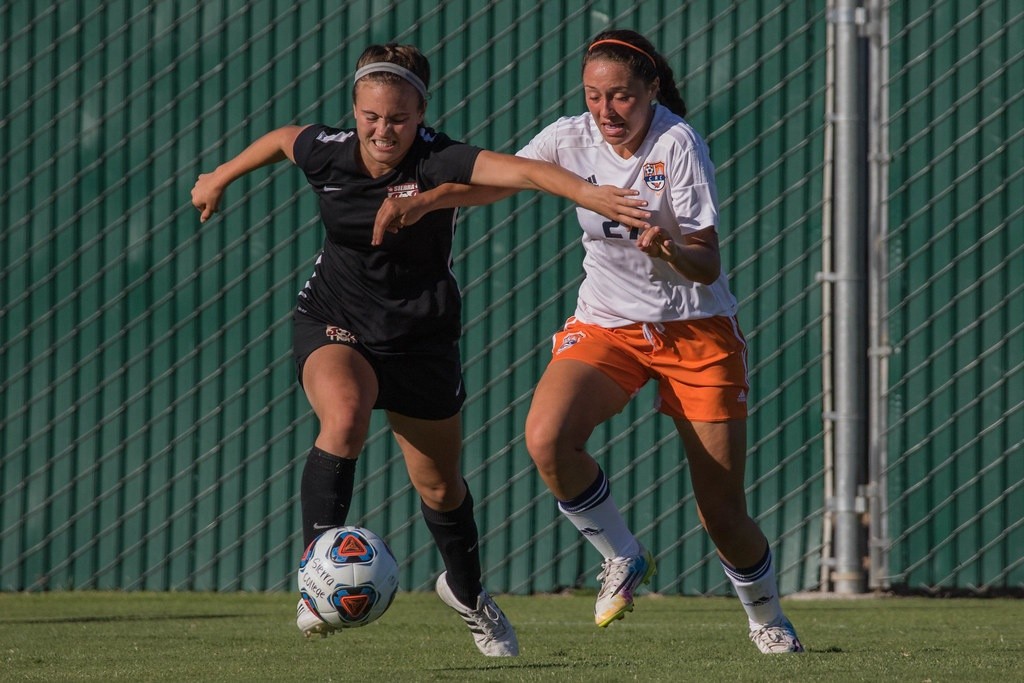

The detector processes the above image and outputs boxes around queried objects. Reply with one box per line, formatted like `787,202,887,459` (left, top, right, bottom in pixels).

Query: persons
186,42,652,656
372,28,807,656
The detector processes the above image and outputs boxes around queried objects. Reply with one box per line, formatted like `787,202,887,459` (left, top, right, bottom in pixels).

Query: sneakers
296,596,342,639
748,613,803,655
594,537,657,628
435,570,519,657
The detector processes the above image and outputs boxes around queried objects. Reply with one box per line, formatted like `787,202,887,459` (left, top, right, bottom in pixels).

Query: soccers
298,525,399,626
